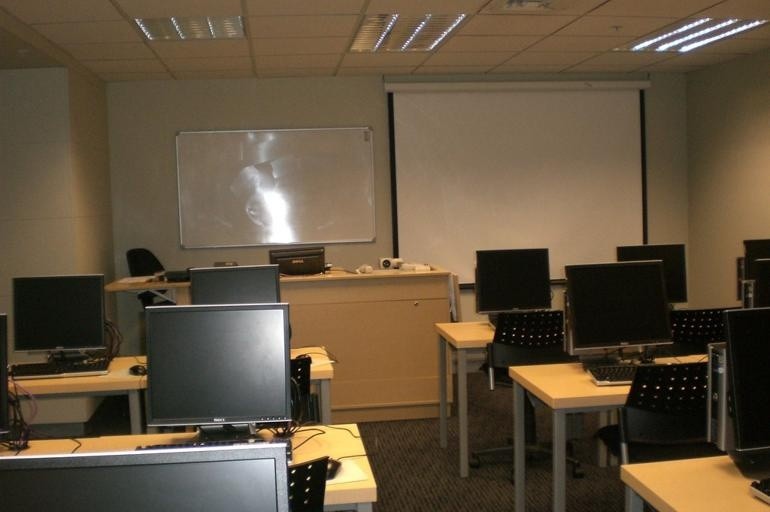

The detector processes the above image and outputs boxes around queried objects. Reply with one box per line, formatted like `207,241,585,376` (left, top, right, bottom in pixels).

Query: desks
506,352,708,511
433,304,690,478
619,455,769,510
0,422,380,511
1,344,335,436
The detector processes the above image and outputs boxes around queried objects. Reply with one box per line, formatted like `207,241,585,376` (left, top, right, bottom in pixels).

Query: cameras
378,255,403,270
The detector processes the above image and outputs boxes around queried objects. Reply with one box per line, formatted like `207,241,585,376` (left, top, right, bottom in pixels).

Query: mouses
130,364,146,375
326,457,340,480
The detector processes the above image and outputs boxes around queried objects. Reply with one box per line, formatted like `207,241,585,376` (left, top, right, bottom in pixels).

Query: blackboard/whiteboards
175,126,376,250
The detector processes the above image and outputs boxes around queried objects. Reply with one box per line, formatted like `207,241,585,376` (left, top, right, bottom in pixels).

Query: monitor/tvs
187,264,281,304
565,259,678,365
268,246,326,275
616,243,688,303
12,274,107,358
740,239,770,302
723,306,770,453
474,247,553,331
1,442,291,512
752,256,770,306
145,302,294,438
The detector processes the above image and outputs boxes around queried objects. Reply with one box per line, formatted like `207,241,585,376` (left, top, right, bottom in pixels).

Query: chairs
123,246,178,356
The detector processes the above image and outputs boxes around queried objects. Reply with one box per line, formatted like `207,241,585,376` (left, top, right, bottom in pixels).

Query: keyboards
586,363,640,386
11,360,111,381
134,439,292,458
748,475,770,506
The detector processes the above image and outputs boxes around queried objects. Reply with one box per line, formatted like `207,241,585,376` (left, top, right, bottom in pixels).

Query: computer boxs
705,340,738,450
